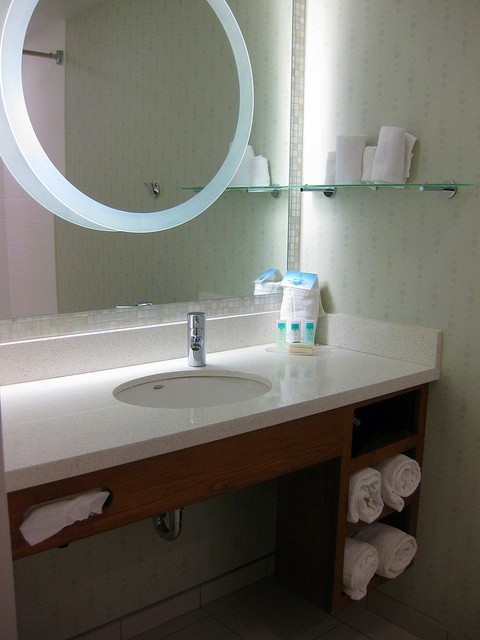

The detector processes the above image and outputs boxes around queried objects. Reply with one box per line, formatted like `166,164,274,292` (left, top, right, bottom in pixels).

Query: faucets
186,312,209,370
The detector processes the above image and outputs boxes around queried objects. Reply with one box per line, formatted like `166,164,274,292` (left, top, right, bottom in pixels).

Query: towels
347,467,384,524
354,523,418,580
340,538,379,602
379,453,423,513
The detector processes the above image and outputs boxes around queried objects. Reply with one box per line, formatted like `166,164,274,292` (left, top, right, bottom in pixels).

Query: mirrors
0,0,306,344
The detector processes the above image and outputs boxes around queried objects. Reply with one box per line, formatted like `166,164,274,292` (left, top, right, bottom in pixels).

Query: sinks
112,369,274,410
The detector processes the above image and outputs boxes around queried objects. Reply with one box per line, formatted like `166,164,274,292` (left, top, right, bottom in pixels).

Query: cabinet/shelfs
182,182,300,200
273,383,429,615
301,179,476,201
